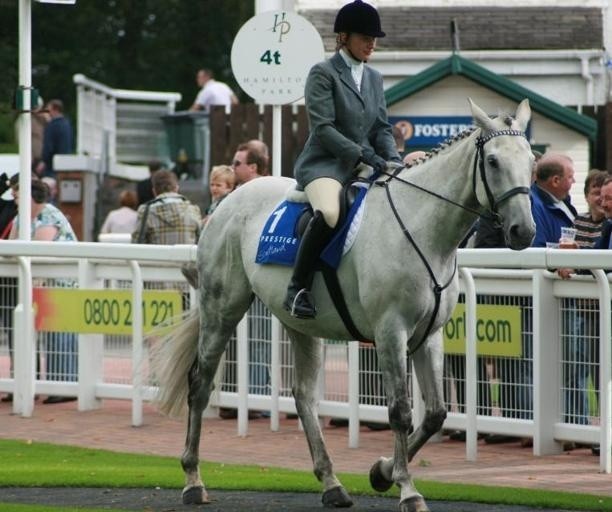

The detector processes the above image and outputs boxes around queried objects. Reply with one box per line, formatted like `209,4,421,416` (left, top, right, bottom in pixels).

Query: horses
129,96,538,512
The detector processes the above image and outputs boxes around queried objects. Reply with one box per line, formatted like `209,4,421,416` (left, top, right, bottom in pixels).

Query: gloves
361,146,388,174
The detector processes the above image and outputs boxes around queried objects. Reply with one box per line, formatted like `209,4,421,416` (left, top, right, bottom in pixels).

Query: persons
0,66,611,457
283,1,405,324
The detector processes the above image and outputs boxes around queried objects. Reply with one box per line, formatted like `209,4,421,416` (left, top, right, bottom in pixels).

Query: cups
544,226,577,249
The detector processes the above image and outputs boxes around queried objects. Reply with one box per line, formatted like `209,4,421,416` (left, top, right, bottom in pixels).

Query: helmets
334,1,385,38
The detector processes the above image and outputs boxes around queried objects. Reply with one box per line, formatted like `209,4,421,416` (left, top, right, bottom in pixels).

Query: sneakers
330,418,520,443
218,406,299,422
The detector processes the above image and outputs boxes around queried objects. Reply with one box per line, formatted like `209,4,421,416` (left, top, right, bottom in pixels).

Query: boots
284,210,337,320
